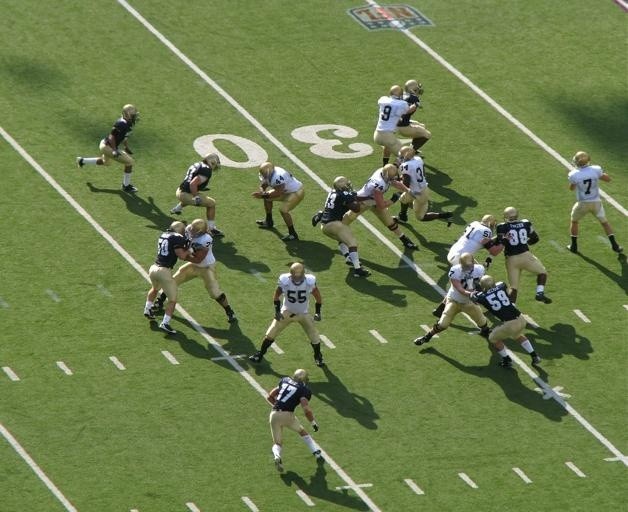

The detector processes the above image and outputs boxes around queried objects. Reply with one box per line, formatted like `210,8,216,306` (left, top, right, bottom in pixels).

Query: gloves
312,424,318,431
313,313,321,321
274,312,284,321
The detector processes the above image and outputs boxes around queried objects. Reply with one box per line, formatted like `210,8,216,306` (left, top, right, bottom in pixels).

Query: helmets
481,214,497,228
460,253,474,272
204,153,220,171
170,218,207,237
122,103,136,119
334,176,351,192
390,80,418,98
294,369,309,383
478,275,496,289
503,206,518,220
289,263,304,281
383,164,397,179
398,146,413,160
571,152,589,166
259,163,273,176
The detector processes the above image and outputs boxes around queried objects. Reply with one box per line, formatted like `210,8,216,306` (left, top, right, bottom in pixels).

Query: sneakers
414,335,429,346
227,314,236,322
256,220,273,226
120,183,138,192
76,157,83,168
210,229,224,236
144,303,177,336
403,241,418,248
249,353,262,362
283,234,298,241
343,257,371,277
534,292,553,304
311,210,322,226
274,455,283,472
447,212,454,228
393,215,408,223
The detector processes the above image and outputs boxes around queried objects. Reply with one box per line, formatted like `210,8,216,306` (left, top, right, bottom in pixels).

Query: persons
143,219,195,334
250,162,305,239
268,369,324,472
412,252,490,346
76,103,141,193
149,218,238,320
310,75,455,278
484,205,551,305
434,212,504,316
168,152,223,235
469,276,542,366
565,151,625,253
249,262,328,366
